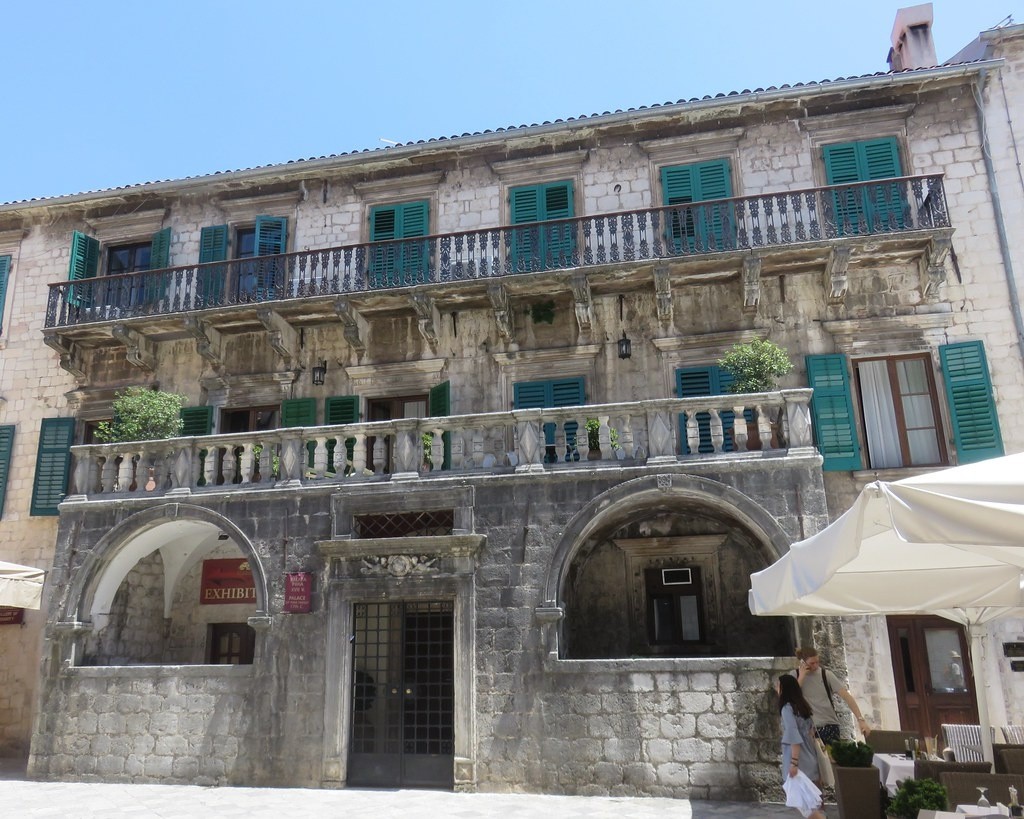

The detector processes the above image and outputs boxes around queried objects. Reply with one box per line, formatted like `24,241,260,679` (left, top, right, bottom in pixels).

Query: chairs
864,721,1024,819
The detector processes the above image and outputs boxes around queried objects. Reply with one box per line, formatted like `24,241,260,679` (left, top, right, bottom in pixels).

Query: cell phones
800,659,805,664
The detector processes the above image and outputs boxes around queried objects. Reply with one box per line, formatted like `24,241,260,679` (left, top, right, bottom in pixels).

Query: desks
955,803,1009,816
873,752,943,800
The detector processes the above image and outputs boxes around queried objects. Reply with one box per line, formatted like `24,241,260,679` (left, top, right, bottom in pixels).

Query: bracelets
858,717,865,720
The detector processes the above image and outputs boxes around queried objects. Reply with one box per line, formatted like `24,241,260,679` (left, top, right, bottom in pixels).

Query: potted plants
719,335,791,450
886,777,947,819
240,447,279,481
573,416,621,459
421,434,436,471
93,390,182,492
831,741,881,819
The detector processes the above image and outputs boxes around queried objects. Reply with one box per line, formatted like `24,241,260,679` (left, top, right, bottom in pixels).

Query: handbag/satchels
813,737,836,791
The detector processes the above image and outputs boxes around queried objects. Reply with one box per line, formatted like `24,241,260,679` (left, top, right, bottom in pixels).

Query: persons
948,651,965,690
778,673,835,819
790,647,870,814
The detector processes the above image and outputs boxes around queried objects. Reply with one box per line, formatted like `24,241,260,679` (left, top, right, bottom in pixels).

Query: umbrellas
748,453,1024,774
0,560,45,611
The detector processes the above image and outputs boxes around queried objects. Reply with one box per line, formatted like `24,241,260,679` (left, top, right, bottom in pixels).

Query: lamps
219,530,229,540
312,364,325,385
616,330,633,358
